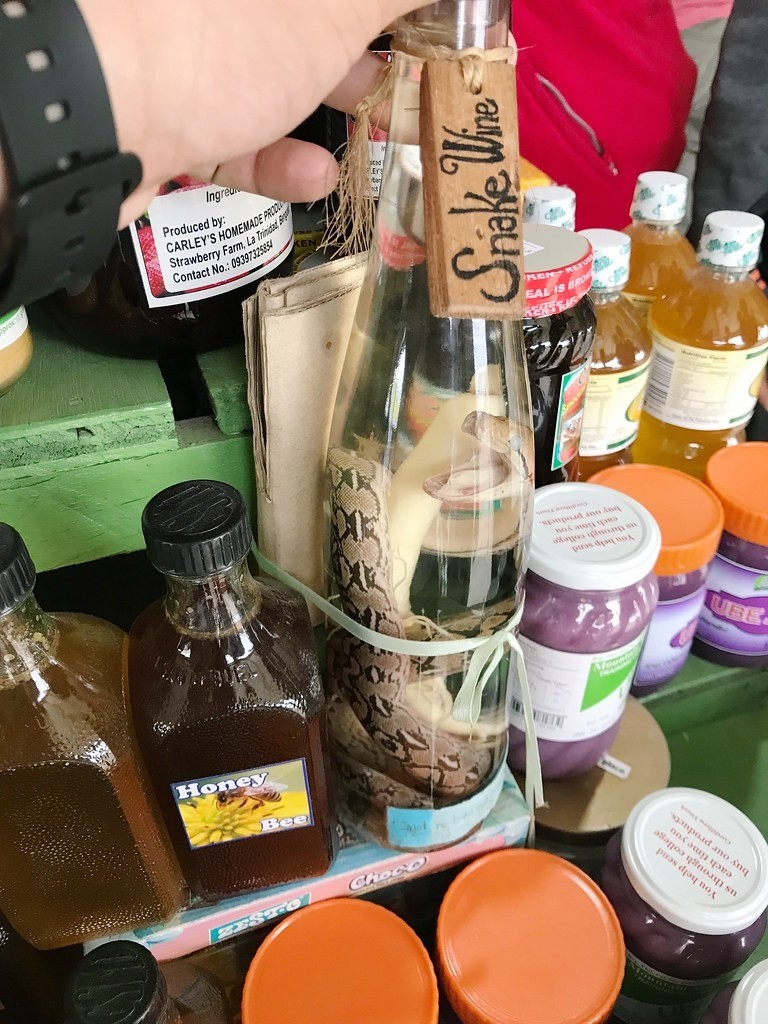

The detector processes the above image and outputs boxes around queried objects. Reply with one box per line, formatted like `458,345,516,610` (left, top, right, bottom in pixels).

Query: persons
0,1,768,316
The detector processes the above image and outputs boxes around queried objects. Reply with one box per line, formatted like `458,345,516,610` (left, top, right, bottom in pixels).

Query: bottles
1,1,768,1024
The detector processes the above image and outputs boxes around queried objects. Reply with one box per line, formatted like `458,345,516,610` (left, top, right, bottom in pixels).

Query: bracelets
0,1,144,317
